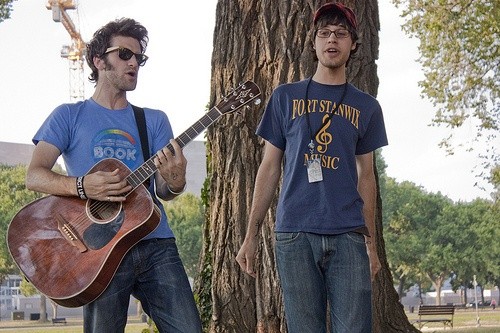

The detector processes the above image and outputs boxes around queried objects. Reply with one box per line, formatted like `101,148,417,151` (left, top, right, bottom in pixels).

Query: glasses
314,27,353,39
95,45,149,68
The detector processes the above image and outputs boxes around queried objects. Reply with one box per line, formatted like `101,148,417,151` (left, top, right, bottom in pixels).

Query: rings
108,197,110,201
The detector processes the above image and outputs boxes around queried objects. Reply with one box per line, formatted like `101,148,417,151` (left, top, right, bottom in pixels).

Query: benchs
412,305,456,330
52,317,67,325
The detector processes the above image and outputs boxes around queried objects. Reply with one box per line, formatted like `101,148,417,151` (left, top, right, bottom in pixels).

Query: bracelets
76,176,87,200
167,184,186,195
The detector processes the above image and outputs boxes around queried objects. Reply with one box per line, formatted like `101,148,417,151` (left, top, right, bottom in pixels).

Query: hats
313,2,359,35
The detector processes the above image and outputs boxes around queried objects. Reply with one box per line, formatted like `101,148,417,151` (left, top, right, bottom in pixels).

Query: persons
235,3,382,333
25,18,201,333
490,298,497,310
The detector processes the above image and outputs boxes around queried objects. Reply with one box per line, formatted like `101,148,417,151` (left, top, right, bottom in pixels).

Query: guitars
6,80,261,308
